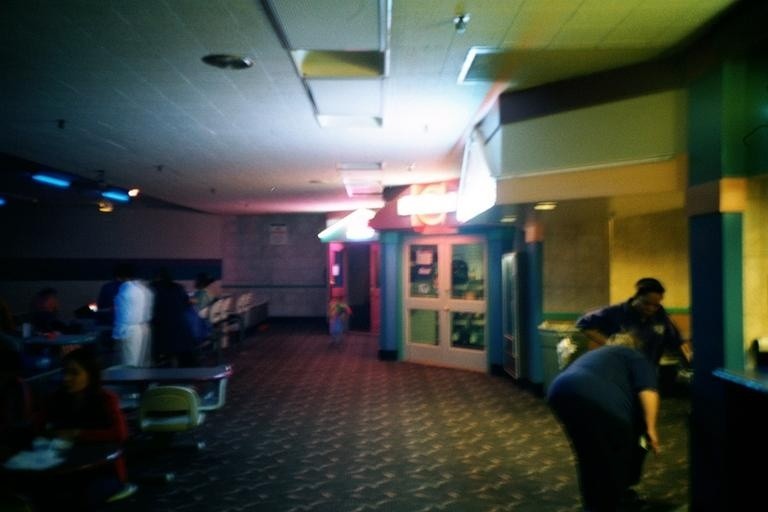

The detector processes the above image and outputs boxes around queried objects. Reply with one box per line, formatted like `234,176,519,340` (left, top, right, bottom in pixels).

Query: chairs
0,282,273,511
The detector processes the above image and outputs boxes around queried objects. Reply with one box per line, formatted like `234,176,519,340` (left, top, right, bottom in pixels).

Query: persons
571,276,692,371
37,349,129,433
22,267,209,366
547,328,665,512
326,287,354,352
